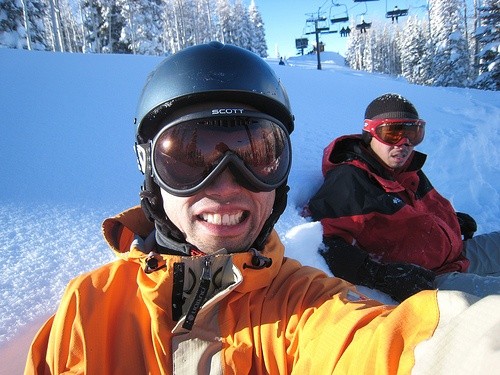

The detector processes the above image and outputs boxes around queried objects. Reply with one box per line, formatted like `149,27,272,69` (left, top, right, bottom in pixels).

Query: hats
361,93,419,146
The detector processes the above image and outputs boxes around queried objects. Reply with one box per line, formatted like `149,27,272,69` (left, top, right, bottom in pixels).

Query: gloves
358,259,437,304
454,211,477,240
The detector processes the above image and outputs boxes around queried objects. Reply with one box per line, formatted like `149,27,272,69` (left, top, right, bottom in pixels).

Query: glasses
149,108,292,198
370,117,426,147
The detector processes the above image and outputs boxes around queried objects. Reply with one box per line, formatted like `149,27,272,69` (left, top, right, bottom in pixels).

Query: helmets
134,41,295,144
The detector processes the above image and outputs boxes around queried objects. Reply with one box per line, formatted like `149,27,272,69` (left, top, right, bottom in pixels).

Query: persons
340,27,346,37
392,5,400,23
278,56,285,65
308,93,499,303
22,42,500,375
360,18,366,34
345,26,350,37
